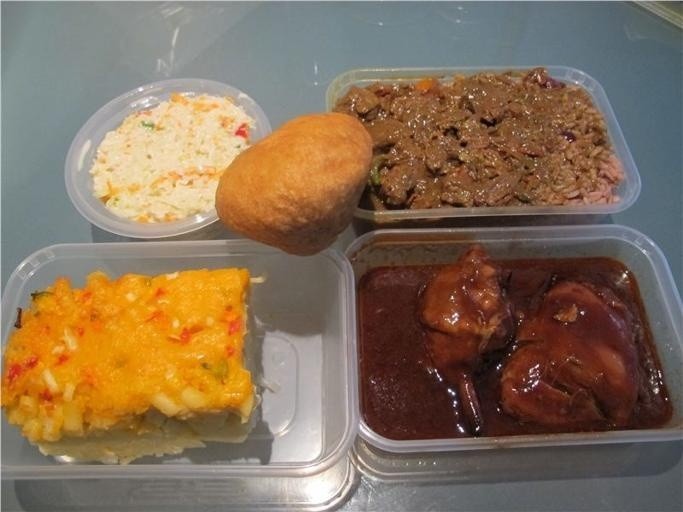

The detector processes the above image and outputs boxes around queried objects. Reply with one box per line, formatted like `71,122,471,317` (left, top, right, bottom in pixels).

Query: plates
63,77,276,238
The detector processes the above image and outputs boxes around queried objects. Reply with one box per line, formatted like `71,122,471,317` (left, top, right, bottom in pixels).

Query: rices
90,94,257,222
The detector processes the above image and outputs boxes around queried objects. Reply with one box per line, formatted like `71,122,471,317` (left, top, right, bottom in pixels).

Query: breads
214,111,374,255
